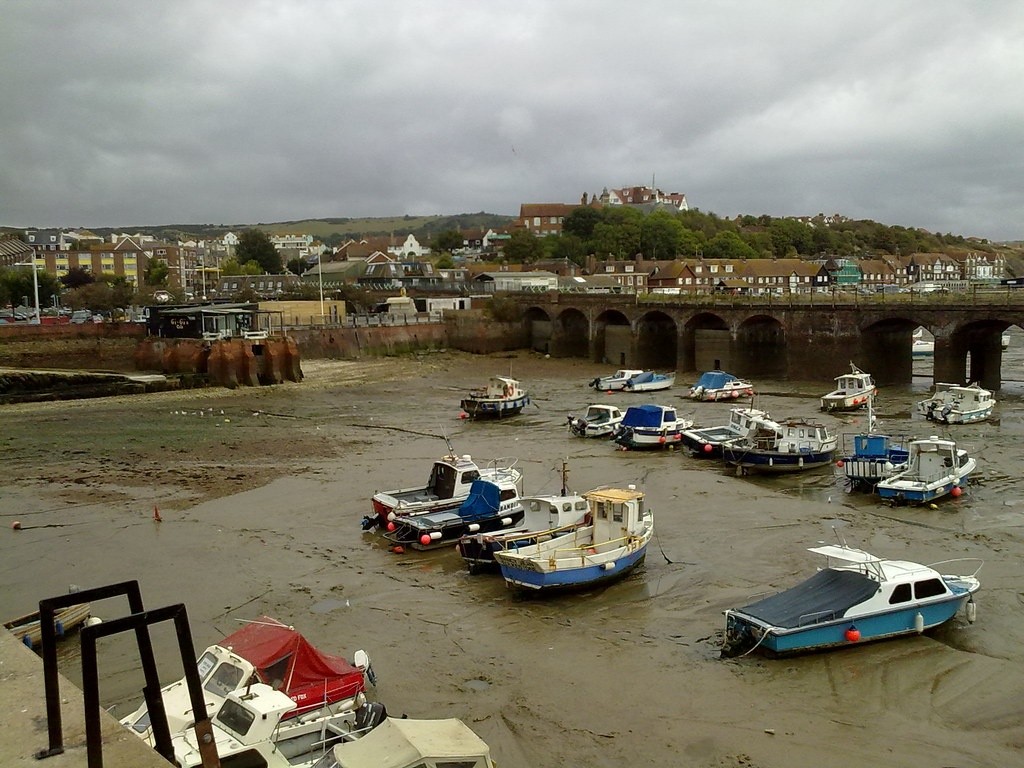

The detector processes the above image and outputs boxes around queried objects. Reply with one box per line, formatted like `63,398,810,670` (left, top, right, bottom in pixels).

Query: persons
401,283,407,297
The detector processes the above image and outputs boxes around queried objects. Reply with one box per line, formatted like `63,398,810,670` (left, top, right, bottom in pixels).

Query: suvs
70,312,94,325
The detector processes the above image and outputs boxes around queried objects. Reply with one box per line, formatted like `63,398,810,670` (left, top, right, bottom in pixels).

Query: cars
902,288,916,295
368,302,392,313
447,243,474,256
746,286,786,302
453,255,468,263
0,299,71,328
814,282,891,295
94,317,101,323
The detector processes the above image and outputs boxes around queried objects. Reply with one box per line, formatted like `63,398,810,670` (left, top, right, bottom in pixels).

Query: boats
678,408,772,457
912,340,935,356
933,386,996,425
918,383,961,416
566,405,628,438
493,487,654,590
840,431,911,483
689,370,754,401
362,456,522,529
118,613,372,752
460,377,531,420
3,584,94,649
719,524,986,660
819,361,877,412
1002,335,1012,350
149,681,405,768
877,436,977,502
588,369,644,390
718,416,839,472
457,493,590,564
610,405,695,450
624,372,676,392
384,475,525,551
294,713,496,768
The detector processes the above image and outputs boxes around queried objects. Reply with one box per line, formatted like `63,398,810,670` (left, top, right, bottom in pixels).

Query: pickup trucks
922,285,949,296
